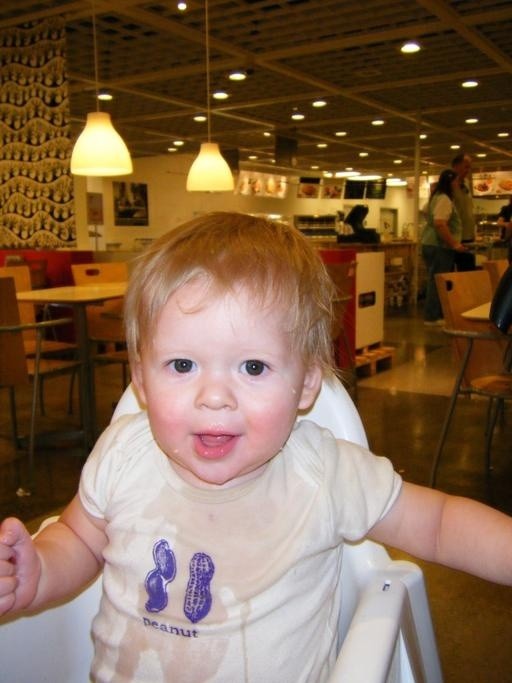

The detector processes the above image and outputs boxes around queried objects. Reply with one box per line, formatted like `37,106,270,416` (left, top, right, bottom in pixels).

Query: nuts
145,539,215,623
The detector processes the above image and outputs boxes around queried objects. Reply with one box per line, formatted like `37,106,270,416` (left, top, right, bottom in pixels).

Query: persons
0,212,512,683
420,152,476,327
497,194,512,239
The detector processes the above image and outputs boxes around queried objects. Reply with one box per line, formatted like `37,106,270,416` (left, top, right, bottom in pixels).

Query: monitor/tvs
344,207,368,223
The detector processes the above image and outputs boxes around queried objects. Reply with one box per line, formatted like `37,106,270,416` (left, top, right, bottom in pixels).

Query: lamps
68,2,134,178
183,1,234,194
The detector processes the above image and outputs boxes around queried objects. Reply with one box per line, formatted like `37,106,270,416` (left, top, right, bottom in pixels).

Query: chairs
0,368,446,682
0,260,133,514
430,259,511,492
